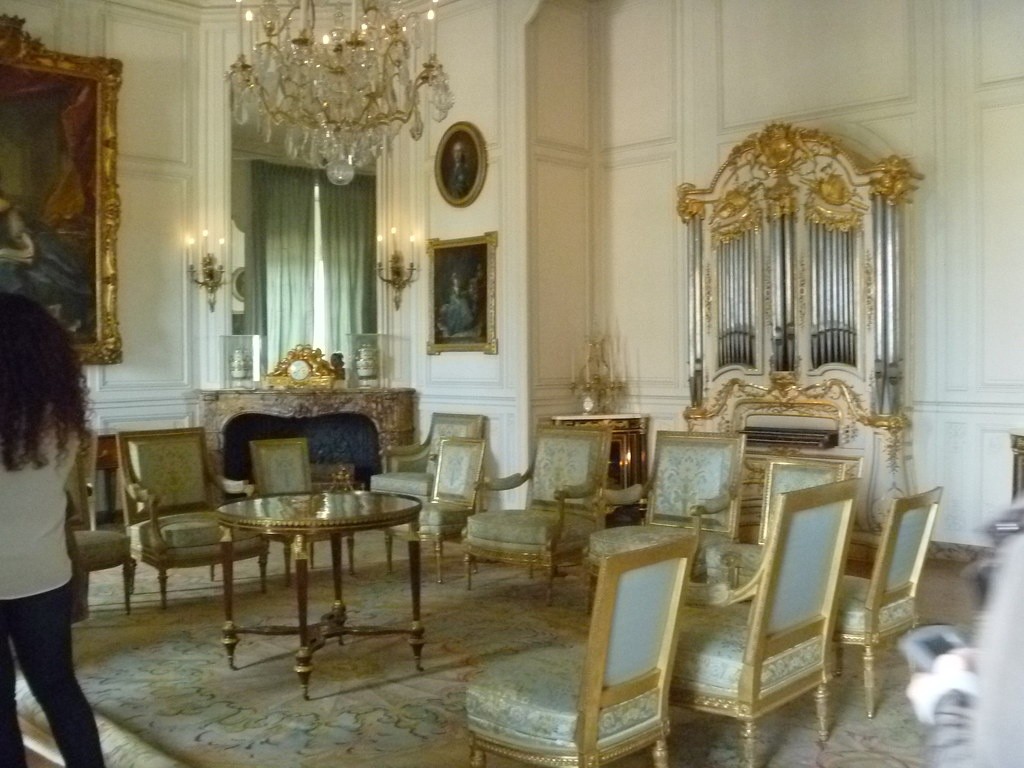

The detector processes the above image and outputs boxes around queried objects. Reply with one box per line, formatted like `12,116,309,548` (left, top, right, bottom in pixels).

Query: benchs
205,410,383,502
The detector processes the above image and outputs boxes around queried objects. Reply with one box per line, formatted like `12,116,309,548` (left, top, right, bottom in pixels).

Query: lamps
186,232,230,312
220,0,455,186
374,227,421,310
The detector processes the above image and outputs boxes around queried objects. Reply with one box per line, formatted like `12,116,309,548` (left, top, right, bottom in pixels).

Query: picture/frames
425,230,498,358
0,13,125,365
434,120,489,208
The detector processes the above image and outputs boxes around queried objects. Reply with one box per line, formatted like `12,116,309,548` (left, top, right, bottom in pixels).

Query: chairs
114,428,268,616
368,412,482,496
62,453,136,616
466,422,613,589
470,535,697,768
588,428,946,767
380,438,486,583
250,434,356,588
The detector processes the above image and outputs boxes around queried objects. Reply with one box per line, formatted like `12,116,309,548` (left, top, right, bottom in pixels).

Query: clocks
265,342,336,391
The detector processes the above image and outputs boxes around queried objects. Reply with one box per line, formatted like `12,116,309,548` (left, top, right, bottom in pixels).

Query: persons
0,292,104,768
908,532,1024,768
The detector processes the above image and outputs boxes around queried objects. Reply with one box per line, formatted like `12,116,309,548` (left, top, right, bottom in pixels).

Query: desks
217,491,423,700
